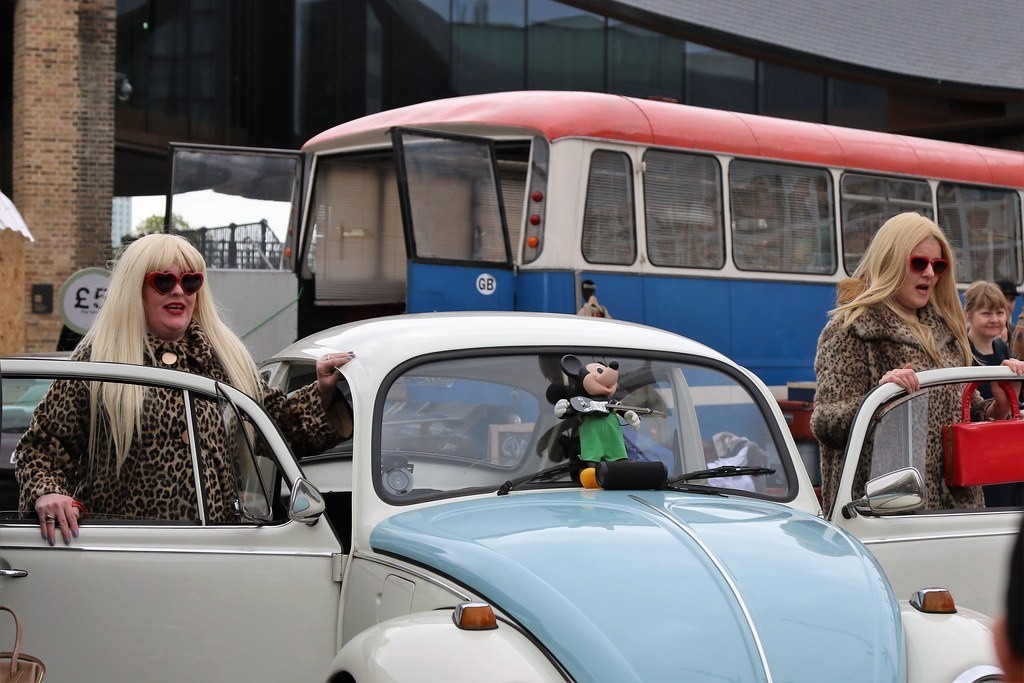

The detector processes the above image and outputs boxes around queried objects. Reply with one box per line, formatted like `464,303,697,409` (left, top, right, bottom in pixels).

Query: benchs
275,488,446,556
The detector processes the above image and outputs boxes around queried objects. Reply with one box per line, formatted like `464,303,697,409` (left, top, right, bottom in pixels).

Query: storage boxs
762,382,823,510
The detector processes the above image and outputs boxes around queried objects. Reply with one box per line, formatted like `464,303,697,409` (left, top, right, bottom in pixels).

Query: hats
995,280,1021,296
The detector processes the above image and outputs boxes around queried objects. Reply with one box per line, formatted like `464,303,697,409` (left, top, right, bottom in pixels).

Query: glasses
907,255,950,277
144,271,205,296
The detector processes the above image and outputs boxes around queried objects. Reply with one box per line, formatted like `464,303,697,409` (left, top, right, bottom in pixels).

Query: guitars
570,395,664,416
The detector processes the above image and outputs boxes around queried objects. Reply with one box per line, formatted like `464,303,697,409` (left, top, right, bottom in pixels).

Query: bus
287,91,1024,523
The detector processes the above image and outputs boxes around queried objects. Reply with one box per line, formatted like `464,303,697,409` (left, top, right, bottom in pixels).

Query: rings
46,515,54,521
326,356,330,360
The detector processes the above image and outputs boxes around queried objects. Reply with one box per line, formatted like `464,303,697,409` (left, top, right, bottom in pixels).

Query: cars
0,312,1023,683
0,352,79,479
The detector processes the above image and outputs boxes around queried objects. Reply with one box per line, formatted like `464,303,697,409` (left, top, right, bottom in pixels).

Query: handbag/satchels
941,381,1024,487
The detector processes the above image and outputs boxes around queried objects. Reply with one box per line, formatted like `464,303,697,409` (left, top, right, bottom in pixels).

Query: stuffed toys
554,354,642,489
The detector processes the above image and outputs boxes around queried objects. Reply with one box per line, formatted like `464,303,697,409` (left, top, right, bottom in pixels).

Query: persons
993,516,1024,683
809,211,1024,519
964,280,1024,507
14,234,355,546
